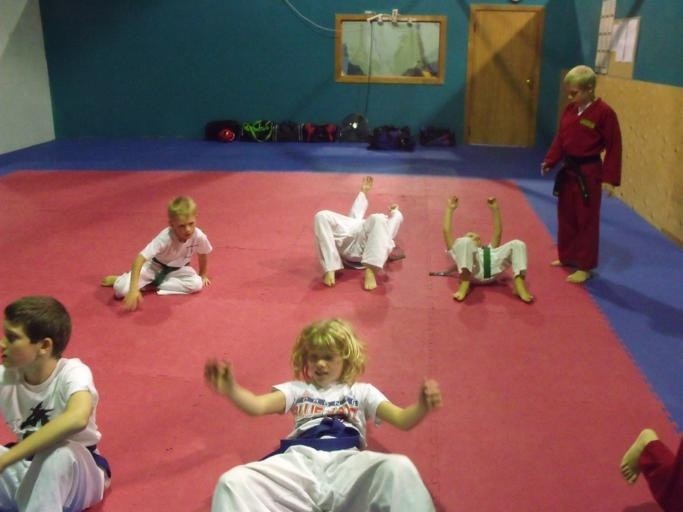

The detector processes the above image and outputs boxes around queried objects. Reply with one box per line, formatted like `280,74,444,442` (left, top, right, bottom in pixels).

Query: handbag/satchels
205,111,374,143
418,125,452,148
367,125,416,151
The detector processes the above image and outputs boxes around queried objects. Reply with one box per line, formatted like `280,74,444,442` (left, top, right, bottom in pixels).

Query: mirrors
334,12,446,84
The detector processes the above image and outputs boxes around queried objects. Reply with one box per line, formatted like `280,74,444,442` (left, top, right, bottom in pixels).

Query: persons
314,177,404,290
1,294,112,512
100,196,212,312
204,317,442,512
619,428,682,511
541,65,620,284
443,195,534,304
